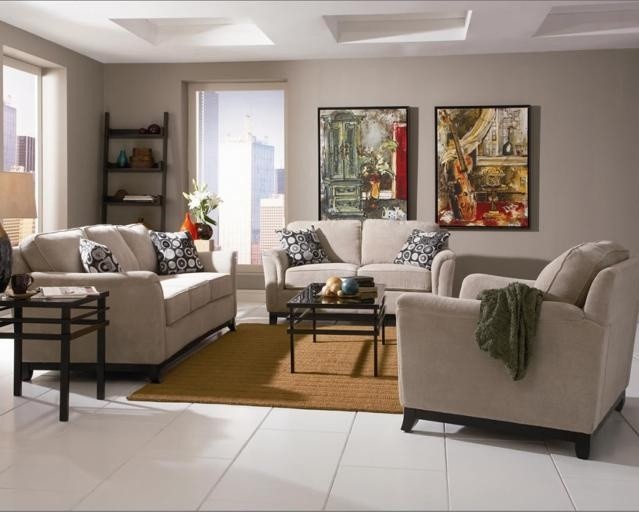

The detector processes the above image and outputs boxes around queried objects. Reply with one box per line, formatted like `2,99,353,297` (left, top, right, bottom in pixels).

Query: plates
6,289,38,300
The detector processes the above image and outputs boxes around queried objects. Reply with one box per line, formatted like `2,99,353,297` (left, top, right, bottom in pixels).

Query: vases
179,213,197,240
117,149,128,167
194,222,213,239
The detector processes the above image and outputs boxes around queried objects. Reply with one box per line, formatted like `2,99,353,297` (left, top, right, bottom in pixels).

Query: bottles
117,149,129,168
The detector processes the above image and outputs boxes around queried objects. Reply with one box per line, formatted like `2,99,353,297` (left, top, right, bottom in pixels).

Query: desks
0,288,109,420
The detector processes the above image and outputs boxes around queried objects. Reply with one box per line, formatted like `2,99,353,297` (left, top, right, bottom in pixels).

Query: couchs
396,240,638,459
261,219,455,325
11,222,238,385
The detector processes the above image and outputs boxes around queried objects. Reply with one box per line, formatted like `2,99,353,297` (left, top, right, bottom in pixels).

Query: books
123,194,160,200
29,285,100,299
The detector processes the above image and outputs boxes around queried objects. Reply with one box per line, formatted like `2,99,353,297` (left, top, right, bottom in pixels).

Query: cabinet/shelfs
102,111,169,231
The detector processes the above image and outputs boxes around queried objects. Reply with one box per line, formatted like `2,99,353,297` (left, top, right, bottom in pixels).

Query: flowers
183,179,223,224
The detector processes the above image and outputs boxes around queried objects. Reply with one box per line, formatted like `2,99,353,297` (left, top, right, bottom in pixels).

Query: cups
11,273,34,293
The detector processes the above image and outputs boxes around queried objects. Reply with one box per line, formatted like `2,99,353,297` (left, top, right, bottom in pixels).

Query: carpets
127,322,403,413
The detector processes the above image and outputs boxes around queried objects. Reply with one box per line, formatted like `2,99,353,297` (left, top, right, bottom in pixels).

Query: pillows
275,224,331,266
79,238,124,273
151,230,204,274
394,228,450,271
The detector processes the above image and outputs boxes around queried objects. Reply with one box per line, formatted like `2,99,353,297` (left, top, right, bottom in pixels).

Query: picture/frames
434,104,529,229
317,106,410,220
